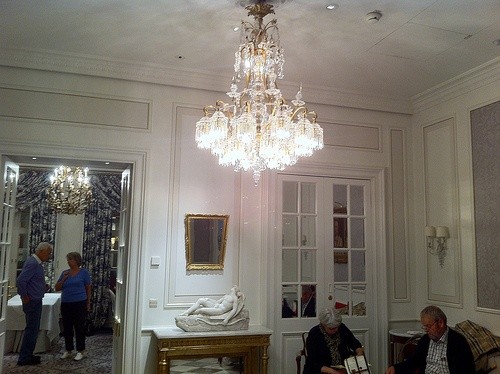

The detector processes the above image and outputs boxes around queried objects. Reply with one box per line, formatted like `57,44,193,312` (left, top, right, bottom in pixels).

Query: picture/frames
333,201,347,264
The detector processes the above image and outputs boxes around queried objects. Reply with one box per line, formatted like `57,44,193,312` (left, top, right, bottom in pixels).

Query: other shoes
60,350,73,359
32,355,41,359
74,352,84,361
17,358,40,365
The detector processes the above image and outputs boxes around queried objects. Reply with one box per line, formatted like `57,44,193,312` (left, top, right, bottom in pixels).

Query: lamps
424,225,450,269
45,164,96,216
193,0,324,187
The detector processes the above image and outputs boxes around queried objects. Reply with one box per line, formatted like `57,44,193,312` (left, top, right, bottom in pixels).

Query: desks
387,327,427,369
6,293,62,354
153,325,274,374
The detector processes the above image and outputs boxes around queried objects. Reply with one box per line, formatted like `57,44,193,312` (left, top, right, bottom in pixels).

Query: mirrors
184,213,230,271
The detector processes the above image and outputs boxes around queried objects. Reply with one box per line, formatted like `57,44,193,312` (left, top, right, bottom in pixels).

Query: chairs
296,332,309,374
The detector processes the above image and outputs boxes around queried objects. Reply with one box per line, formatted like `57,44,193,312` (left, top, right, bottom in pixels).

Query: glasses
422,320,440,330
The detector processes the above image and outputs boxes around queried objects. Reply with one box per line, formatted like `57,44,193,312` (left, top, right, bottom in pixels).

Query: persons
54,251,92,360
385,305,476,374
300,284,317,317
178,285,243,326
16,242,52,363
302,306,372,374
